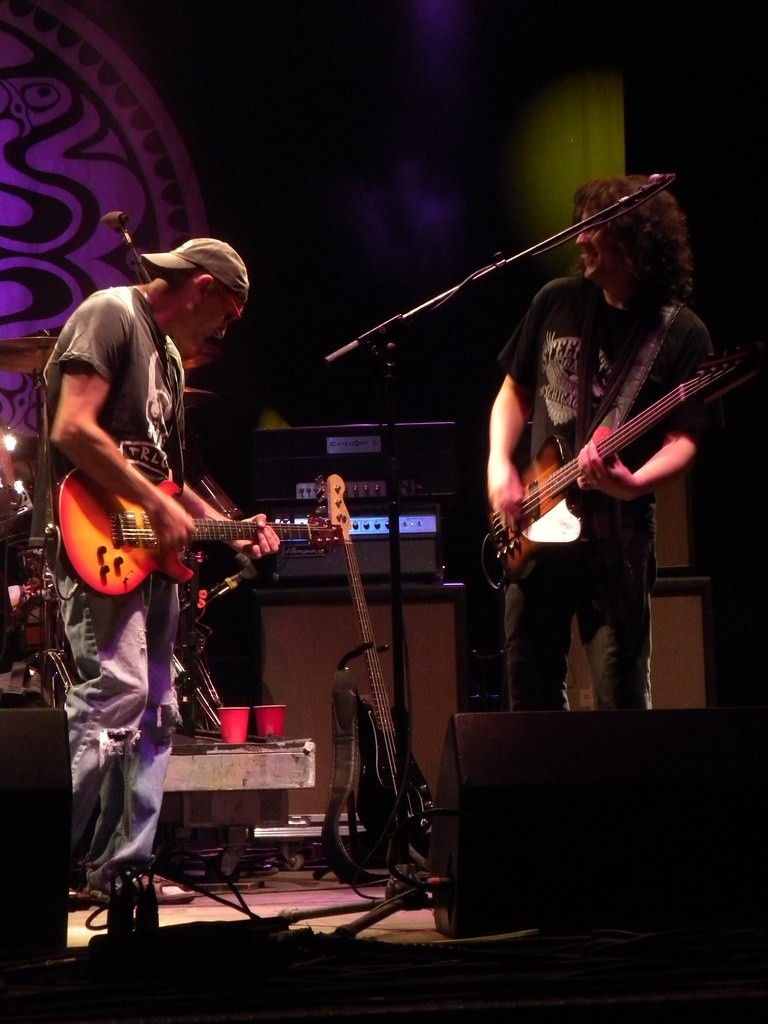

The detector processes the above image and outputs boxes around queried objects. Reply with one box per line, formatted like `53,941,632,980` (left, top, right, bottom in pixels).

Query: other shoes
68,887,97,913
84,869,204,905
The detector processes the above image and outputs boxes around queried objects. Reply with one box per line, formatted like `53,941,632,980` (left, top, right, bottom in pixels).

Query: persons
42,237,283,909
486,177,725,714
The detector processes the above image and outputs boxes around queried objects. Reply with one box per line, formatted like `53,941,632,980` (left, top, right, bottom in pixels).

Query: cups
254,704,287,737
217,707,253,744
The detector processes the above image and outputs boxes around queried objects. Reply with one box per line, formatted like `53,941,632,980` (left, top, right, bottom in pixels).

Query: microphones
649,173,677,185
101,212,131,228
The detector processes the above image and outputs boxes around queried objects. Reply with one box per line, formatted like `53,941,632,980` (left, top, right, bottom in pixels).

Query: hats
140,238,250,301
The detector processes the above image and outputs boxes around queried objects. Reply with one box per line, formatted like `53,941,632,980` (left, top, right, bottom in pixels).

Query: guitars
314,469,435,842
47,461,343,597
487,336,768,582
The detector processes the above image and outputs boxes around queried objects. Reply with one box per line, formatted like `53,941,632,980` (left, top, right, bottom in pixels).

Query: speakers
0,708,72,949
428,706,768,938
